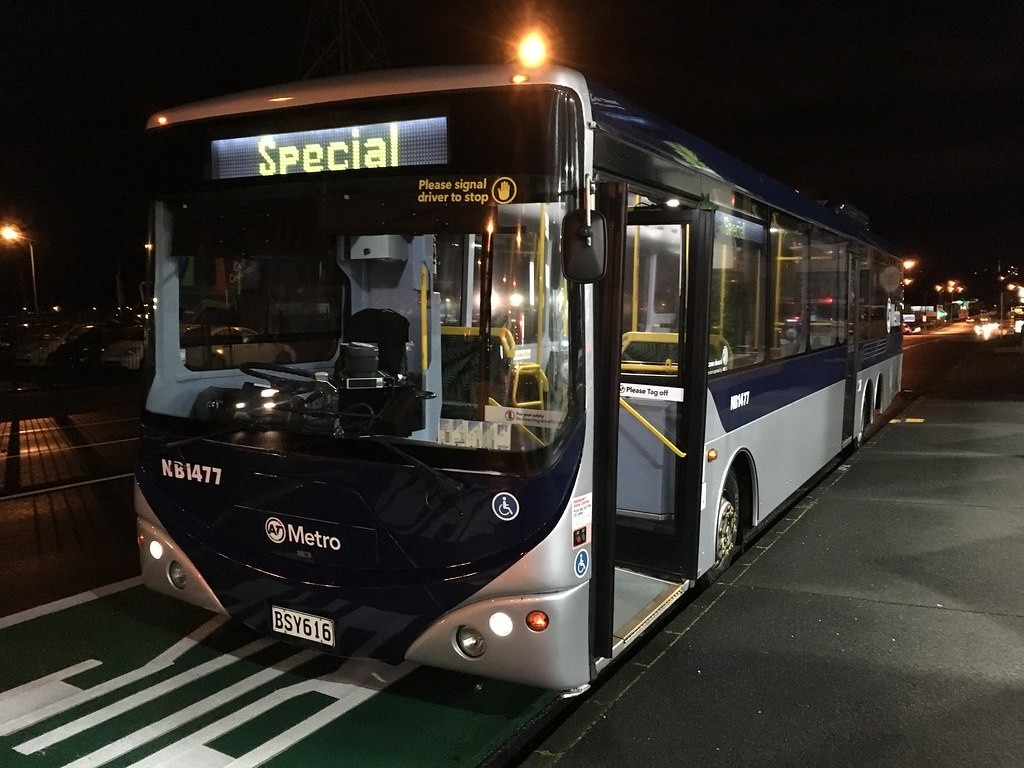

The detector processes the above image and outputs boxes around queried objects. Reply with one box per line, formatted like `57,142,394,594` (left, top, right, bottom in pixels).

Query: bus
132,59,906,699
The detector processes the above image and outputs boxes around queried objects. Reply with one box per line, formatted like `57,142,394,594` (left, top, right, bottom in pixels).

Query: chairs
333,306,410,380
440,326,515,422
622,330,734,376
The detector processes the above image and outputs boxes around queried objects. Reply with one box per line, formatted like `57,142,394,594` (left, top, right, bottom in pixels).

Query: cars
100,325,210,375
50,325,142,378
184,326,296,371
14,321,93,369
